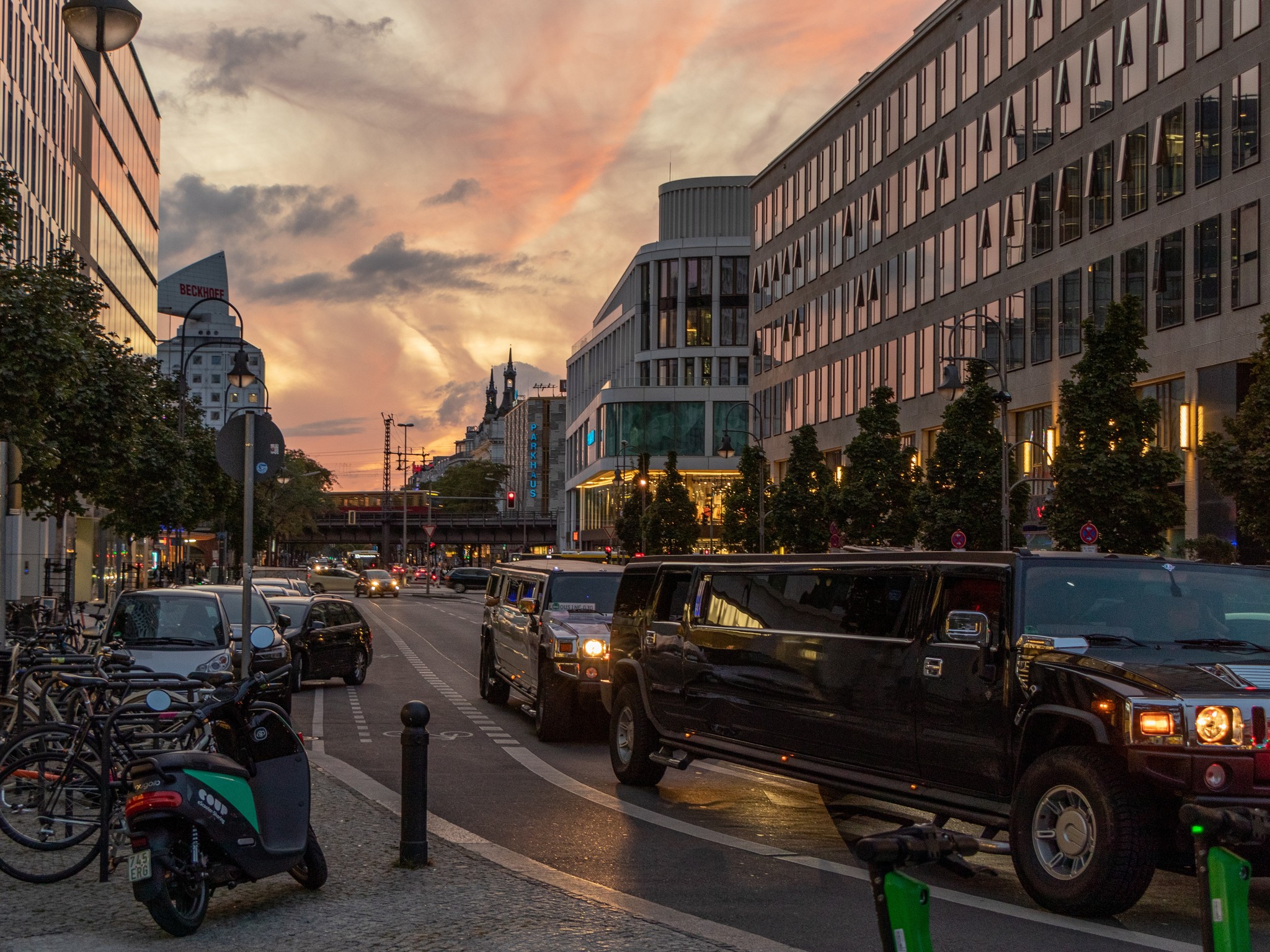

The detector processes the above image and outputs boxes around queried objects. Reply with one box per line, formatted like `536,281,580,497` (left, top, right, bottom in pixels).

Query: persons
433,564,442,588
407,564,414,587
151,558,243,588
438,561,470,572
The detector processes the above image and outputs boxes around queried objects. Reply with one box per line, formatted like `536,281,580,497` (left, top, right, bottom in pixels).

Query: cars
354,563,449,598
297,557,360,593
445,567,519,593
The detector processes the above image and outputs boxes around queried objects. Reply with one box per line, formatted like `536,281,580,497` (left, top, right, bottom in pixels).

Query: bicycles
0,595,235,886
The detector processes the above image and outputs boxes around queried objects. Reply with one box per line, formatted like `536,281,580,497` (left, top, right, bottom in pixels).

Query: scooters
120,625,328,935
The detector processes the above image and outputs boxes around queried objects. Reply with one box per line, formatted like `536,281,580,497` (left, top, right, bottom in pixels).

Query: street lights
716,402,764,554
937,314,1010,552
612,445,646,556
269,471,321,568
397,423,414,588
485,478,526,554
175,298,255,585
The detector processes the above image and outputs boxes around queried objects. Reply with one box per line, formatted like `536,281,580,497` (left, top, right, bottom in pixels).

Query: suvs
607,547,1270,923
237,577,373,693
480,559,626,742
93,589,252,707
167,583,292,717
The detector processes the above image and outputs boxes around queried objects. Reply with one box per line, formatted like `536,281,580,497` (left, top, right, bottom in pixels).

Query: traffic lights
429,543,436,555
507,492,516,510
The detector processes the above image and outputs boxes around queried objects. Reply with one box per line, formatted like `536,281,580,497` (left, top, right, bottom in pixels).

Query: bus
348,550,380,574
507,551,630,567
321,490,443,517
